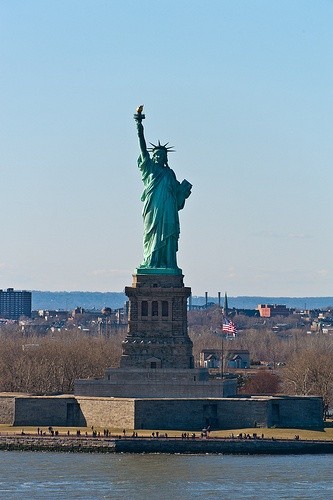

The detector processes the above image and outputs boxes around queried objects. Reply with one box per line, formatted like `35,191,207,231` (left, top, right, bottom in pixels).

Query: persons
21,424,301,441
137,121,191,270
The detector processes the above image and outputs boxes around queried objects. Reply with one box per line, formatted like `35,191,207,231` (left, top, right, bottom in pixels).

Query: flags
223,316,237,334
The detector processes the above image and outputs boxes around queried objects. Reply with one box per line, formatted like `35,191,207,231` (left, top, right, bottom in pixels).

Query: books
179,178,193,199
